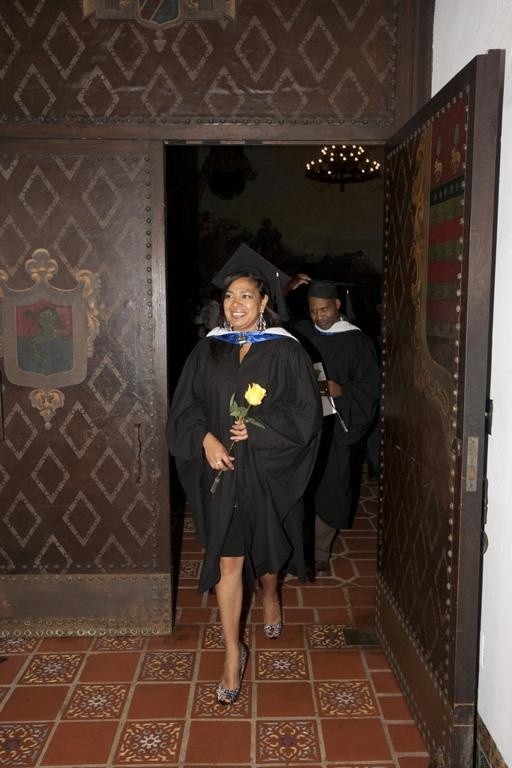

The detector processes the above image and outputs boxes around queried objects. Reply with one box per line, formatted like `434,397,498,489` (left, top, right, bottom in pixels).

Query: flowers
206,377,273,494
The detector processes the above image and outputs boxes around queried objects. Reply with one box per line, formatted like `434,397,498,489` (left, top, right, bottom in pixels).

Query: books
312,361,338,419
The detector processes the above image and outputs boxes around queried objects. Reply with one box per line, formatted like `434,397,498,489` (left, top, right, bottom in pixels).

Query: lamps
302,141,388,198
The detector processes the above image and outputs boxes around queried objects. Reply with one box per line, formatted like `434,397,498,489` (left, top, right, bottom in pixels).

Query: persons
252,216,285,263
163,240,326,705
286,269,382,568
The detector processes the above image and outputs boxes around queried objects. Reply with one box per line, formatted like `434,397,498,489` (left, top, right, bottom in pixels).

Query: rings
217,458,222,465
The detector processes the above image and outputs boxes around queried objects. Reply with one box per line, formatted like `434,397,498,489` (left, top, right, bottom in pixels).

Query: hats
304,278,359,299
211,243,293,301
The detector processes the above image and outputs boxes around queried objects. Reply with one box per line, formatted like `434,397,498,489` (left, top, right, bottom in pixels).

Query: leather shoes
264,620,282,640
314,559,333,577
215,642,249,706
